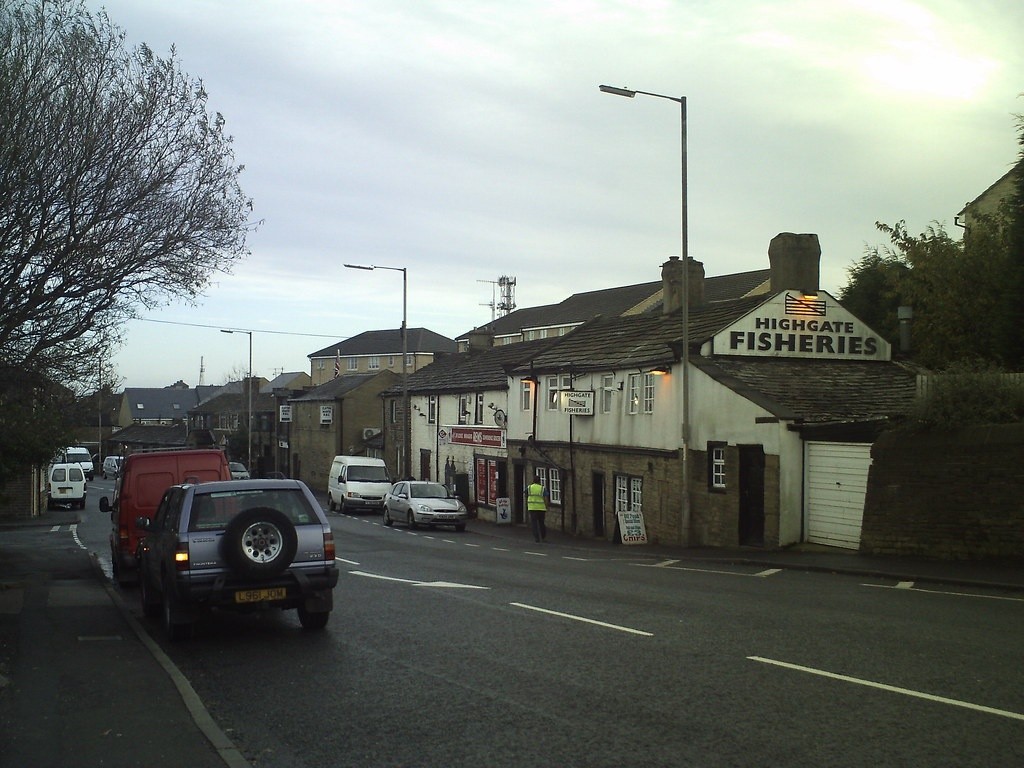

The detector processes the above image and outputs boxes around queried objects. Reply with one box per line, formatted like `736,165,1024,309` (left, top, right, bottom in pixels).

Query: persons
525,476,549,543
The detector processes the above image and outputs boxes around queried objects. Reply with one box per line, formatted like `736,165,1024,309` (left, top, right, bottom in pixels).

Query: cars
382,481,467,533
229,462,250,480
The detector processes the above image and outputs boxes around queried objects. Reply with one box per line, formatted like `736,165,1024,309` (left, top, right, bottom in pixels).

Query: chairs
195,497,217,524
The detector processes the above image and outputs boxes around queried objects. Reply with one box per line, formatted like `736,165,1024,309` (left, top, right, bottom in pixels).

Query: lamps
494,410,507,426
650,367,669,375
803,292,819,298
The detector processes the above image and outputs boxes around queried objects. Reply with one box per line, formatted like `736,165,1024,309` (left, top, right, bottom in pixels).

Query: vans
327,455,392,515
64,447,94,481
98,444,237,582
48,462,87,510
103,457,126,481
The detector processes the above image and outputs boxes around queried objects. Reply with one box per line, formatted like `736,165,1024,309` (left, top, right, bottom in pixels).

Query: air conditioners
362,427,381,440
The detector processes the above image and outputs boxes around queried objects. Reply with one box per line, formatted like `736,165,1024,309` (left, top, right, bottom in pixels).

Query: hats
533,476,540,483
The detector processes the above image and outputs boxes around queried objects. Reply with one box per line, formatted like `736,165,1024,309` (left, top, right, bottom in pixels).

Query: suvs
135,472,340,646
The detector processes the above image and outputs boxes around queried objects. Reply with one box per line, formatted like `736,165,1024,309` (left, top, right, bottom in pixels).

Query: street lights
598,83,692,551
220,329,252,474
344,263,411,478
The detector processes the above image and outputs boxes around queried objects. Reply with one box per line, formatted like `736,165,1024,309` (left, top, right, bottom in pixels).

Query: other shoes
535,537,539,542
541,534,545,539
